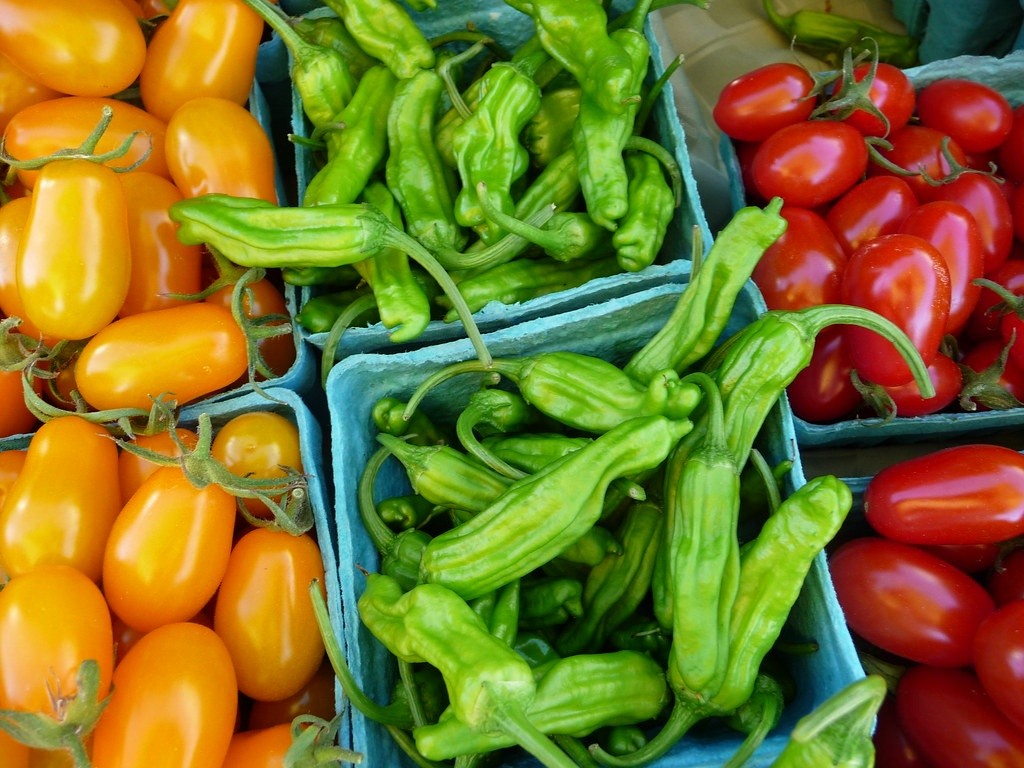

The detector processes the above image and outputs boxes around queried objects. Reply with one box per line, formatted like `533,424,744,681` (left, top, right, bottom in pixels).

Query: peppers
171,0,932,768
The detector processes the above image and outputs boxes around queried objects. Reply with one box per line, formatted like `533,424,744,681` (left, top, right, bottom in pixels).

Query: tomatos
710,35,1023,768
0,0,363,768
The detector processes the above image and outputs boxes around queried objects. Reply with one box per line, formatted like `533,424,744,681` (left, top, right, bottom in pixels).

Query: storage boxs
3,0,1024,768
